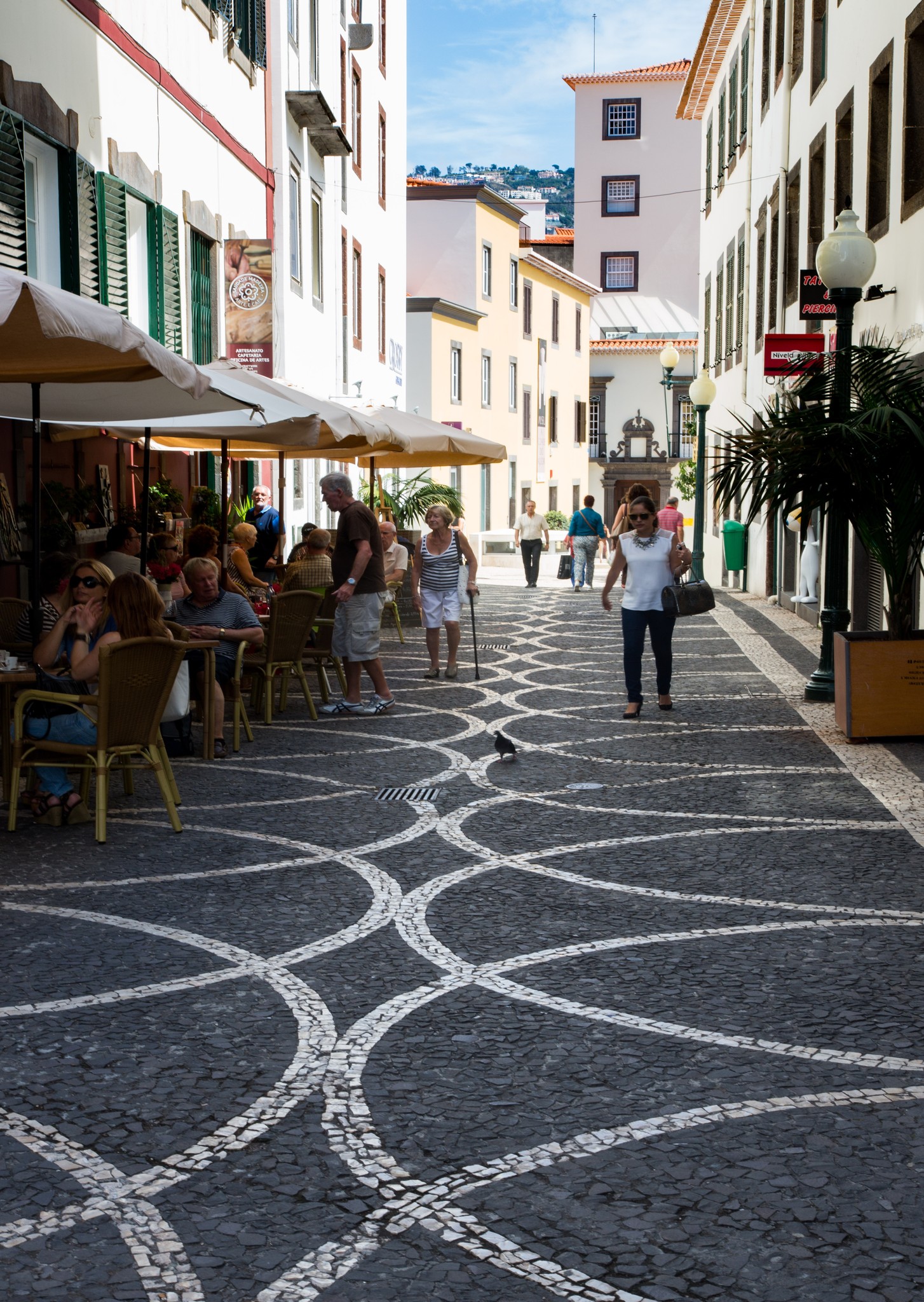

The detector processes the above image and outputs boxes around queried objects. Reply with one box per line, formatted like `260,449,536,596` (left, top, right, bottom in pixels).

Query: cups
162,600,178,621
5,652,10,657
5,656,18,670
0,650,6,660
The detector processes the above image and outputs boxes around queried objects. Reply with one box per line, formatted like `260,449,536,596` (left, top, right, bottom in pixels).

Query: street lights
689,368,716,583
804,208,877,704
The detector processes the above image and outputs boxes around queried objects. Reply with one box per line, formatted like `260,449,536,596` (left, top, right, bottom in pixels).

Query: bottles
269,584,275,596
266,586,273,607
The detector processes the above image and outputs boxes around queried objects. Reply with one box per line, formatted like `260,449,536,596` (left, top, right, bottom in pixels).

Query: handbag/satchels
557,555,571,579
661,562,715,618
454,529,480,605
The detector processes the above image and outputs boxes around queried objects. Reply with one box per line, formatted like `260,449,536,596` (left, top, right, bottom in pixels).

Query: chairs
381,557,412,643
9,638,187,849
282,587,350,710
242,589,324,728
0,596,38,662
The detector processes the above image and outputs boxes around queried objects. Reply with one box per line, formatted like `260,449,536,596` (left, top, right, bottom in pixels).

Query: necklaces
633,529,660,551
430,527,448,545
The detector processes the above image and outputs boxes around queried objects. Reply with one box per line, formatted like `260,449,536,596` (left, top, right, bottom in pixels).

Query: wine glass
255,591,263,607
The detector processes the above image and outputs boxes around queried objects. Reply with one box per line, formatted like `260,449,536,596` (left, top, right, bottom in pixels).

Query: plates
0,666,27,673
0,660,5,663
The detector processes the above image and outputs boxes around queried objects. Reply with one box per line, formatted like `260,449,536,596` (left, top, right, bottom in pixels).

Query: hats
301,523,317,537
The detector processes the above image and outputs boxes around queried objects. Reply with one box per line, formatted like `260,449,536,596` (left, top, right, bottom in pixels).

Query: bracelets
611,541,615,545
546,542,549,544
412,594,420,598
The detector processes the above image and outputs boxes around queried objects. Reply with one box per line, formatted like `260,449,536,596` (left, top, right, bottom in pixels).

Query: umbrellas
0,265,263,655
343,356,510,518
79,356,417,579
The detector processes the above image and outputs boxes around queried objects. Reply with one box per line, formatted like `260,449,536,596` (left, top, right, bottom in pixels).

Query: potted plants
694,314,923,742
354,466,466,547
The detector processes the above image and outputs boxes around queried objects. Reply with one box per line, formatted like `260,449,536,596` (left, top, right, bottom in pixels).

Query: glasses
131,534,141,542
214,539,219,546
163,545,178,552
69,575,104,589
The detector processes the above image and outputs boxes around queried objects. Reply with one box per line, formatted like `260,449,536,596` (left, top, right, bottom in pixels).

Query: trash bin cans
719,518,746,571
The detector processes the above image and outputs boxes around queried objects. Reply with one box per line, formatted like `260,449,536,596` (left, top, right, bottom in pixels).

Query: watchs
681,561,692,570
467,580,476,585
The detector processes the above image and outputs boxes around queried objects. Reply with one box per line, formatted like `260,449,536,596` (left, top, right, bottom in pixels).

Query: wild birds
491,731,518,763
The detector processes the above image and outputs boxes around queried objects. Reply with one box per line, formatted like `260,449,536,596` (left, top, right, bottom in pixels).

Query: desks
170,637,222,763
0,663,100,802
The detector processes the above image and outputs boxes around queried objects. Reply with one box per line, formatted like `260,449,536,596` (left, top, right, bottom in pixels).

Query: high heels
34,793,61,827
30,796,47,810
658,694,673,710
62,790,91,824
622,697,643,718
21,790,36,805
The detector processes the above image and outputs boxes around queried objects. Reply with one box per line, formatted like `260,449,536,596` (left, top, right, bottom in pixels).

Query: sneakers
587,586,594,591
444,663,458,677
525,584,531,588
214,742,229,757
356,693,395,715
531,582,537,587
424,667,440,677
574,581,580,591
319,698,364,714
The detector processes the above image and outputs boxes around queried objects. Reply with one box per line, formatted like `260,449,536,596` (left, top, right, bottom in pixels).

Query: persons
512,500,551,589
597,523,611,564
563,532,587,590
0,558,409,834
410,504,480,678
600,496,692,720
565,495,608,592
610,482,652,601
657,496,685,545
